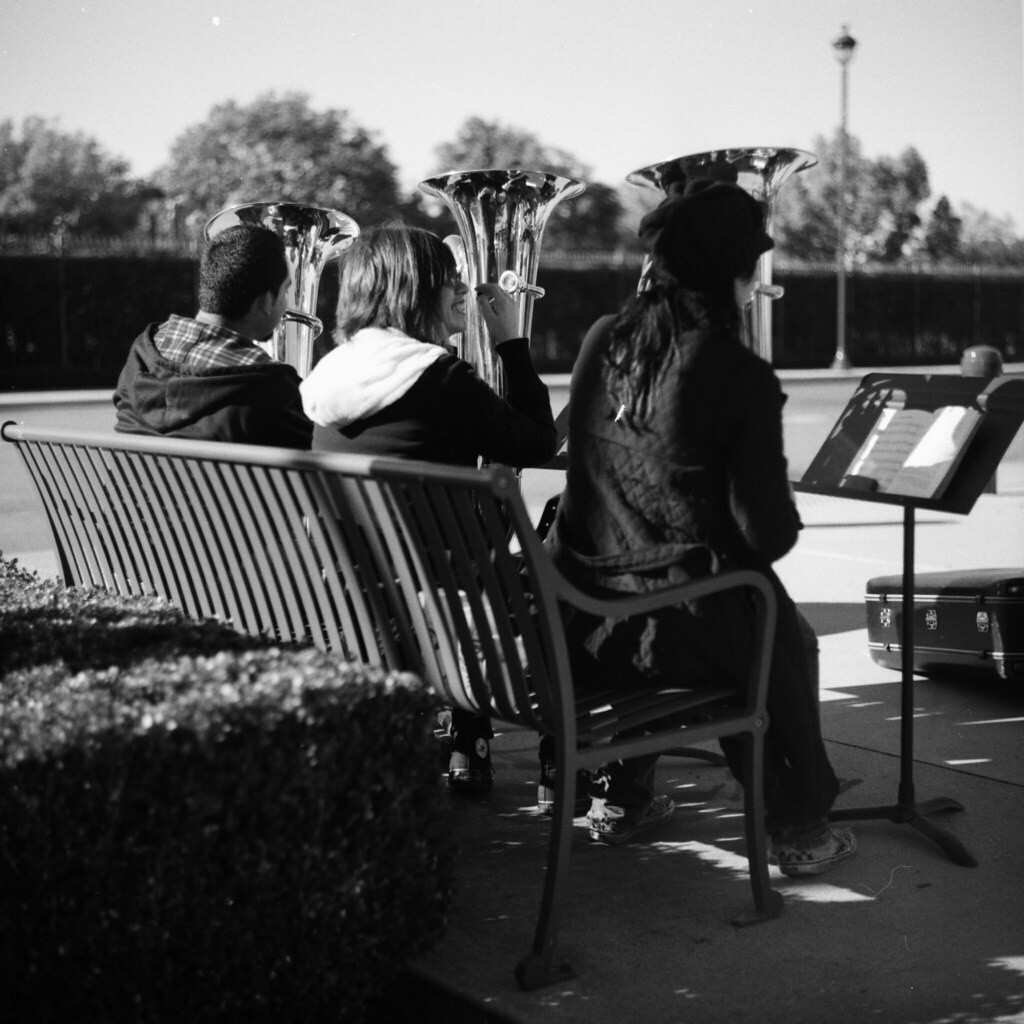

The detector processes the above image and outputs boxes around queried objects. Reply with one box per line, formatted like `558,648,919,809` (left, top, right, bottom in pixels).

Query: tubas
622,144,818,366
198,204,362,381
416,169,587,399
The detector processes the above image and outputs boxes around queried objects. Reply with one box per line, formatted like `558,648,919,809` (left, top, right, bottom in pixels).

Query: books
842,395,986,503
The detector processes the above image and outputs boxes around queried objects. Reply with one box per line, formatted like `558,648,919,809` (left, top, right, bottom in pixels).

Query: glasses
430,271,463,287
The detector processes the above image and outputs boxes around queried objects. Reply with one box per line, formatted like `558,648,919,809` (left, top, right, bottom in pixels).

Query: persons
302,222,676,849
561,179,864,878
114,222,309,460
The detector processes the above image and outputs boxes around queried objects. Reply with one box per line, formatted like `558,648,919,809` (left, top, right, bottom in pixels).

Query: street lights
830,20,858,367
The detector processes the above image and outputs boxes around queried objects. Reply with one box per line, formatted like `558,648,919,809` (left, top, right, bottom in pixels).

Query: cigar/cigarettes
489,289,507,304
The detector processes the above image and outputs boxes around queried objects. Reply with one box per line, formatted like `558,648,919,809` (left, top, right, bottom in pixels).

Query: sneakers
449,749,494,786
586,795,676,842
777,823,858,876
536,774,585,815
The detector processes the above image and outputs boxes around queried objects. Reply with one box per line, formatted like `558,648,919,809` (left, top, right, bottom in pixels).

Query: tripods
789,368,1024,868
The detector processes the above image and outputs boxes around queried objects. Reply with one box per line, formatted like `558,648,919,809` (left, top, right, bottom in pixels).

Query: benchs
0,420,785,990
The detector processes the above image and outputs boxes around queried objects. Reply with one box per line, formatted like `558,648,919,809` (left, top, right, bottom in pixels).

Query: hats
636,179,775,254
